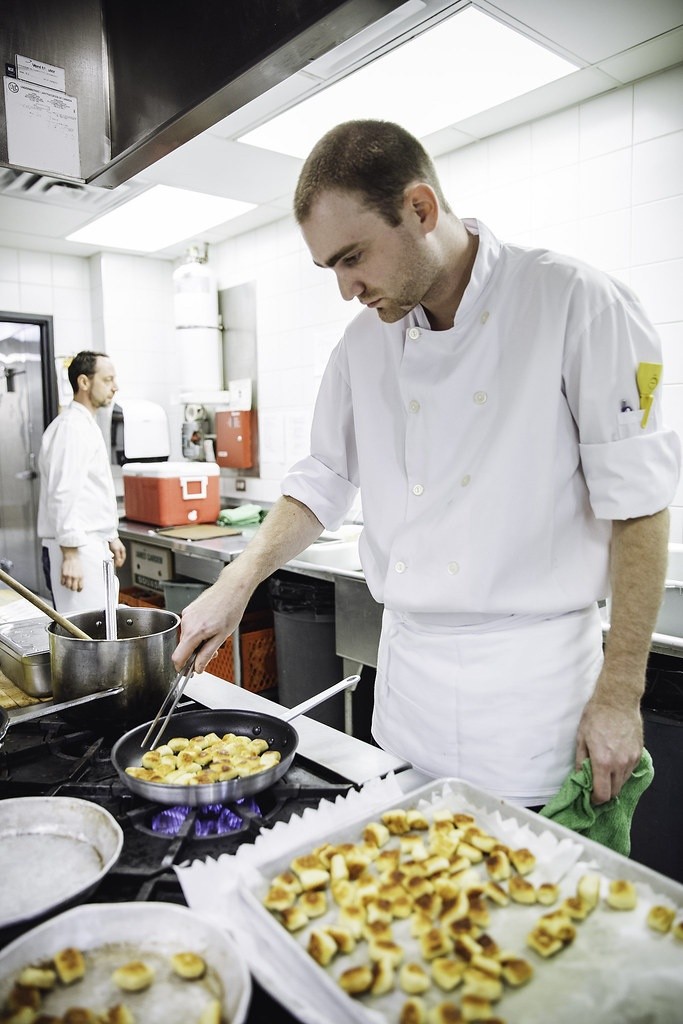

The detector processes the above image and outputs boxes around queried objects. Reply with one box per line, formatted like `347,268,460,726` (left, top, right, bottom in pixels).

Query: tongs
139,641,205,749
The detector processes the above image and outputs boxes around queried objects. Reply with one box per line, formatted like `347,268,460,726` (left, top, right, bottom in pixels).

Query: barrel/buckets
122,460,221,525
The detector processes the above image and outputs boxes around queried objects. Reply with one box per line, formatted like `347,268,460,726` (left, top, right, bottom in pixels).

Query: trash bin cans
265,575,369,732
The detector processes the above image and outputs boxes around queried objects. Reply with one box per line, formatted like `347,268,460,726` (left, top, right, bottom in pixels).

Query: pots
45,576,181,710
119,674,363,804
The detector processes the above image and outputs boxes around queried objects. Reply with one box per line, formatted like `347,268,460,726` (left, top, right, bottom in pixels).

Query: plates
0,795,136,927
0,903,252,1024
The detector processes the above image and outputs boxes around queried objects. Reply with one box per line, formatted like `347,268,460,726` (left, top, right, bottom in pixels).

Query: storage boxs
118,586,277,693
121,462,219,526
215,407,256,468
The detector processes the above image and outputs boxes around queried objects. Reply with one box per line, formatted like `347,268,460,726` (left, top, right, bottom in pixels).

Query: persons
37,352,127,616
173,121,683,817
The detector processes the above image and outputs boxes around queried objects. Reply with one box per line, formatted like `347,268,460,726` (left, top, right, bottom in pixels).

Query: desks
116,517,298,688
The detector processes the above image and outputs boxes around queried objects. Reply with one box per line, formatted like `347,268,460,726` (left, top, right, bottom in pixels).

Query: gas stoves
0,670,434,1024
